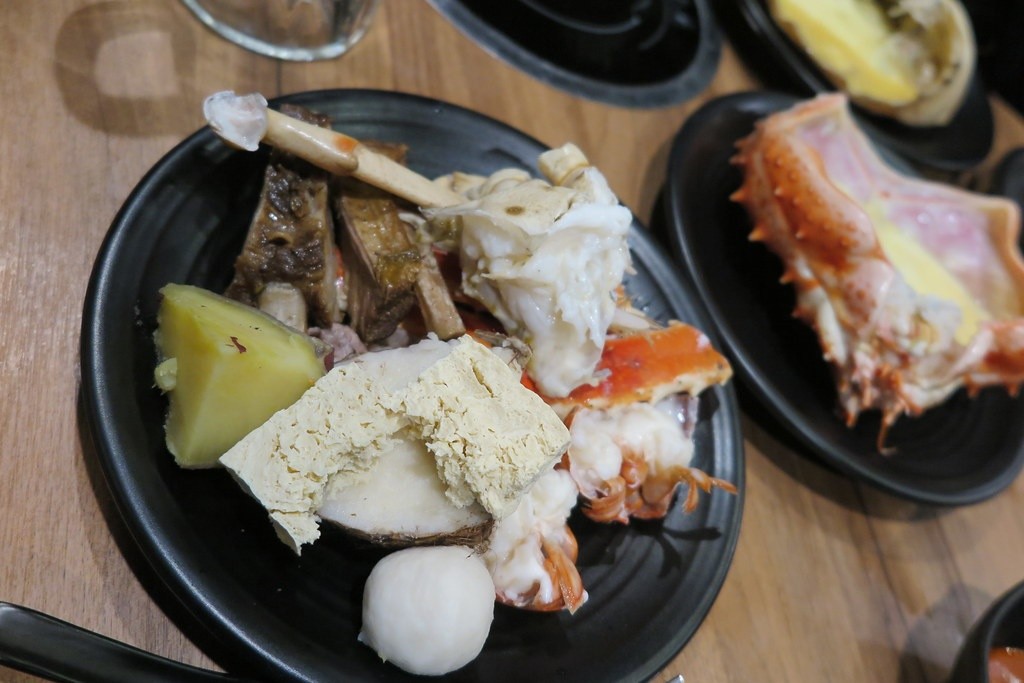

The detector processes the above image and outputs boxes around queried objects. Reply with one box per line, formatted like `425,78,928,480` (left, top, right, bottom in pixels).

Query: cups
953,581,1024,683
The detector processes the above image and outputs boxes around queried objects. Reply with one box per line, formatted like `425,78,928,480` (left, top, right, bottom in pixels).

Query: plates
423,0,722,112
651,93,1024,524
708,0,997,169
88,89,746,683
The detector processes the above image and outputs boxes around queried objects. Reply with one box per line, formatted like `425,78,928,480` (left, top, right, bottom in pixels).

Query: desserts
361,546,497,674
456,146,632,400
